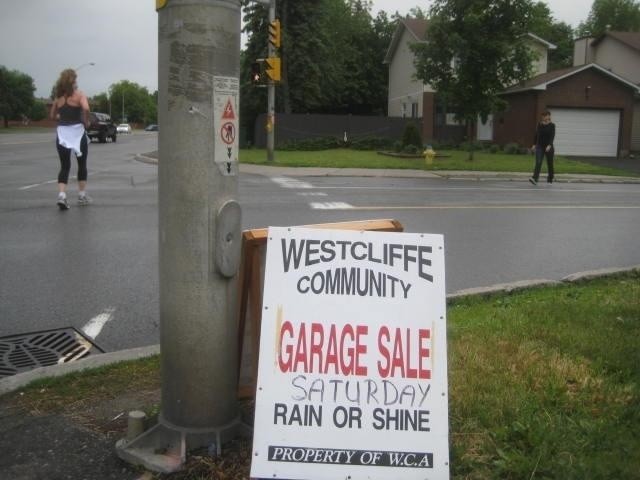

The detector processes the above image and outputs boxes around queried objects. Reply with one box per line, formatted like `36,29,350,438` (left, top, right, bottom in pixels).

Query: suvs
86,112,117,144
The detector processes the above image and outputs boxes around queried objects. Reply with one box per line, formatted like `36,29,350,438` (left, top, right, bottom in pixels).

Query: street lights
75,61,95,75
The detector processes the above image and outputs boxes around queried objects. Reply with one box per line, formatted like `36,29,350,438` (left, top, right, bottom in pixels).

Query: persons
49,68,93,211
529,110,555,186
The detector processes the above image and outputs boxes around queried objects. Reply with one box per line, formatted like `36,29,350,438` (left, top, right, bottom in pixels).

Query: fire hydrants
422,144,435,164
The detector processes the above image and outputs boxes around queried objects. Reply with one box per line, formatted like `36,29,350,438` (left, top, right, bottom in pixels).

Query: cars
117,123,131,134
145,124,159,131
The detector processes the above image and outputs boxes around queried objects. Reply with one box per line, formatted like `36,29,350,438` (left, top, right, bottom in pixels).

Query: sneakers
529,177,537,185
57,196,70,209
78,196,88,206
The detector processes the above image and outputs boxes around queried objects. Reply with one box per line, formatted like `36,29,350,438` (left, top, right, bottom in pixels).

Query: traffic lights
251,60,266,86
269,19,280,48
263,58,280,83
